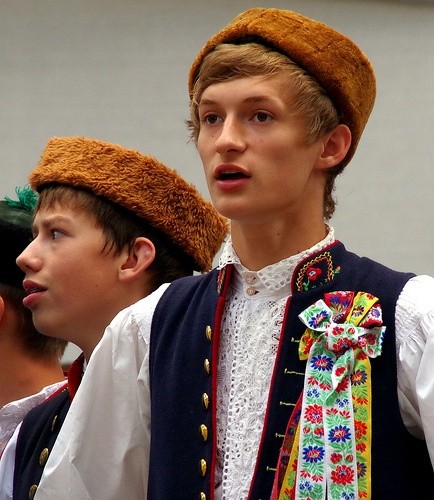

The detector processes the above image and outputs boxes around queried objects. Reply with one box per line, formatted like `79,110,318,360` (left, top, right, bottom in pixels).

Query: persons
0,202,69,460
34,7,434,500
0,134,231,499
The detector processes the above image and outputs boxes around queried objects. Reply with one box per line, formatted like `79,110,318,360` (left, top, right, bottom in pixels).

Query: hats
188,7,375,173
29,137,230,271
